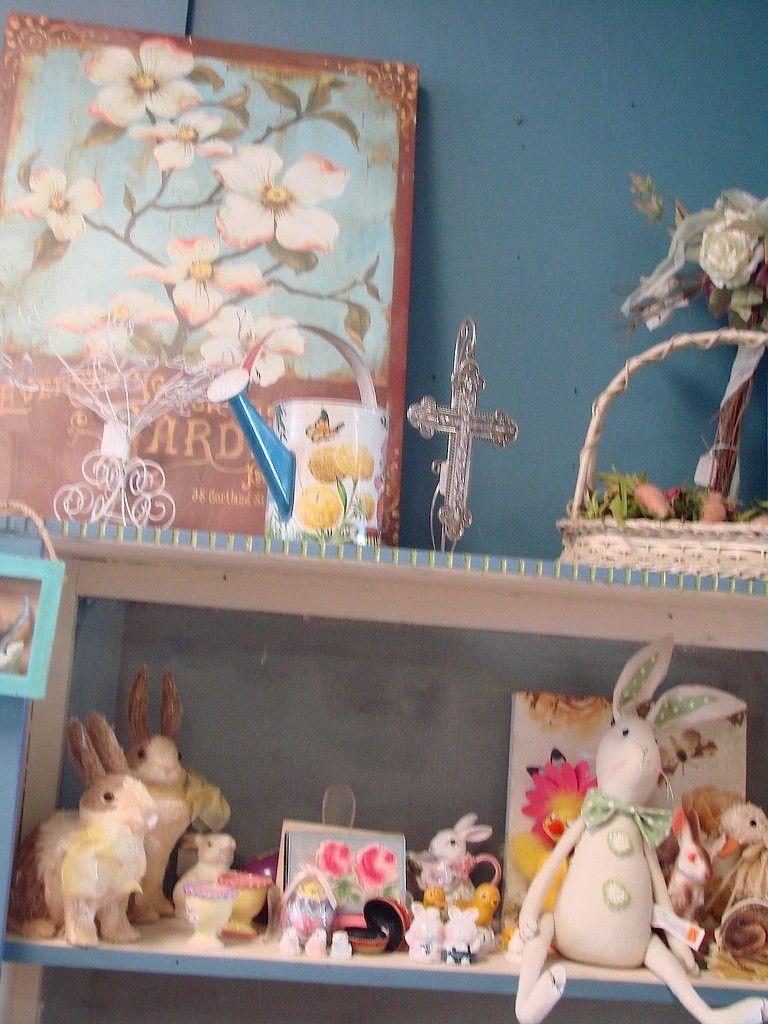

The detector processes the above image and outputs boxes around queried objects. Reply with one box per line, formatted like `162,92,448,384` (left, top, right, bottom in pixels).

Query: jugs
205,323,390,548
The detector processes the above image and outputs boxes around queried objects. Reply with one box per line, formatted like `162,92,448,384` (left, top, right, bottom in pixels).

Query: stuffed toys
668,801,768,973
10,667,196,950
515,644,768,1024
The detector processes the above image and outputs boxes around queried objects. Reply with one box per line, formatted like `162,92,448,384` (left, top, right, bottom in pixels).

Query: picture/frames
0,13,419,547
0,554,65,699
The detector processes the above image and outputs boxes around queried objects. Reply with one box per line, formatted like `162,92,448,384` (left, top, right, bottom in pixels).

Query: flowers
618,170,768,509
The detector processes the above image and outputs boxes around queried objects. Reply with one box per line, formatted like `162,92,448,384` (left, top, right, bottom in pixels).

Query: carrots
632,484,727,523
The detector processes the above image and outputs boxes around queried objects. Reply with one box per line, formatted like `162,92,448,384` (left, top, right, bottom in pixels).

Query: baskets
555,326,768,580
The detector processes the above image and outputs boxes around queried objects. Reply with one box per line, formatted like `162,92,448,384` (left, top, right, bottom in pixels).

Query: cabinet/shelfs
0,514,768,1024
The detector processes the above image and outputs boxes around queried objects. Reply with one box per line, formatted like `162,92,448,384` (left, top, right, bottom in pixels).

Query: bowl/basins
362,897,410,954
342,925,388,955
